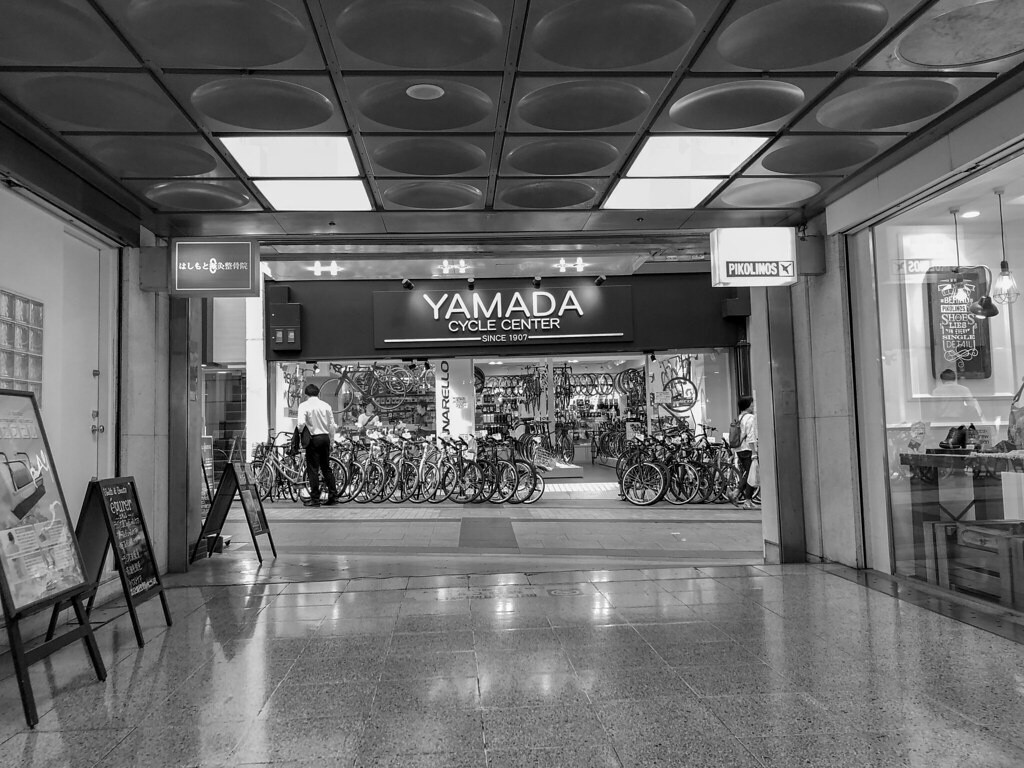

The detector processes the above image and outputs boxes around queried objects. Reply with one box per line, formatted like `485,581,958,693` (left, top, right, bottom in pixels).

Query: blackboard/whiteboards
54,475,163,611
198,462,270,538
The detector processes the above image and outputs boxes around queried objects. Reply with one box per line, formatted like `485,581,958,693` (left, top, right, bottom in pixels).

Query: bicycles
209,354,761,506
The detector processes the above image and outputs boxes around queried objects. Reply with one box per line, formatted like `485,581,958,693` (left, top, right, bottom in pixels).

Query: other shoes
751,485,760,499
986,439,1017,453
736,499,747,504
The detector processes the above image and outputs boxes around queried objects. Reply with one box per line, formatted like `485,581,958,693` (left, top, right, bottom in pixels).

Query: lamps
951,205,972,306
306,360,320,373
951,265,999,319
417,358,430,370
402,357,416,370
643,350,657,363
993,183,1021,305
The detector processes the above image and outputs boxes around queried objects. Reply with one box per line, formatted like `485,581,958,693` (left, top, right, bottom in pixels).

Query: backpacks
729,413,747,448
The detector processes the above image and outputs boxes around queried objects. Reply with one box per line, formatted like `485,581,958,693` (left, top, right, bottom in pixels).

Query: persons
357,403,379,427
928,369,975,417
414,400,433,431
298,383,338,507
728,396,758,510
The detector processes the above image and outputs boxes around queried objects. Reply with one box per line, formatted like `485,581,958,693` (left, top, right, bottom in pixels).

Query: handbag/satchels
1007,382,1024,450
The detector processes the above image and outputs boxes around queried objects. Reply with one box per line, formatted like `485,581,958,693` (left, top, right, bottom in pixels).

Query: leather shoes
939,425,967,449
303,499,321,507
965,423,981,449
321,499,336,505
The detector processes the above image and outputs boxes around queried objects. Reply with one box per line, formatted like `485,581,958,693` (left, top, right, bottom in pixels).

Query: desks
899,452,1024,581
